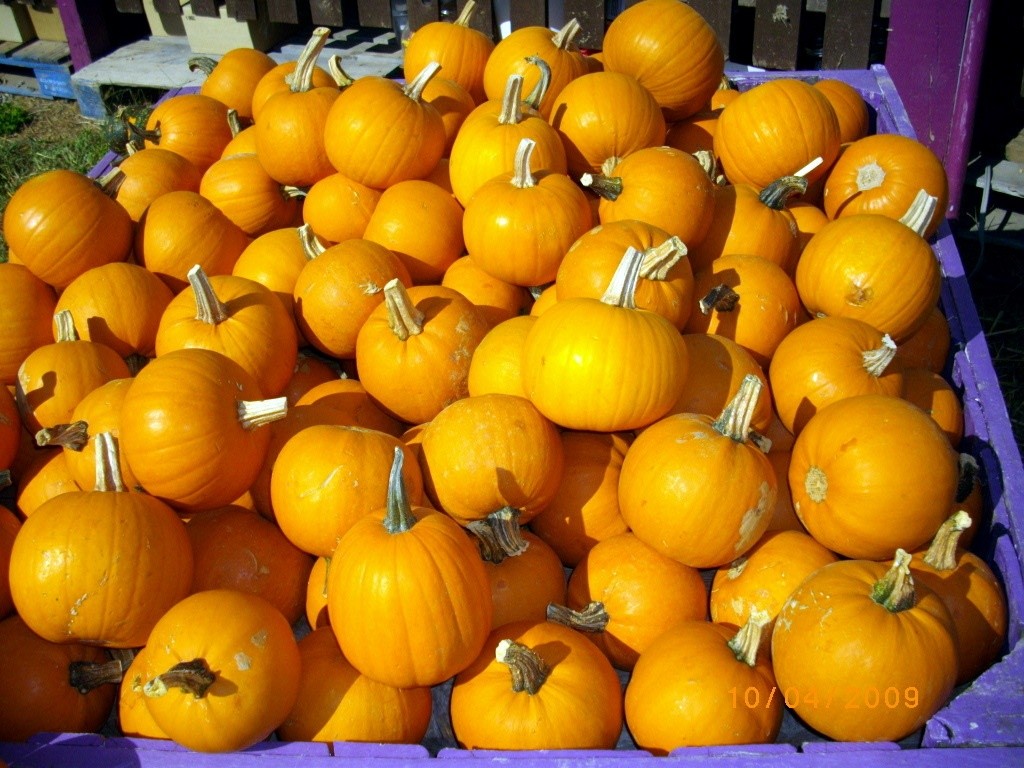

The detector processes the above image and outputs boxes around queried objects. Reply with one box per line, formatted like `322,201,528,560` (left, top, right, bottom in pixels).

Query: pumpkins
0,0,1005,768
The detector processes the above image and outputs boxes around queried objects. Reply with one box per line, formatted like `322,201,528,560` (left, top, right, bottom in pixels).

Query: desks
70,22,403,89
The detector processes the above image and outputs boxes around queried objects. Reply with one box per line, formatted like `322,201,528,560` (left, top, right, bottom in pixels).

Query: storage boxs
0,64,1024,767
